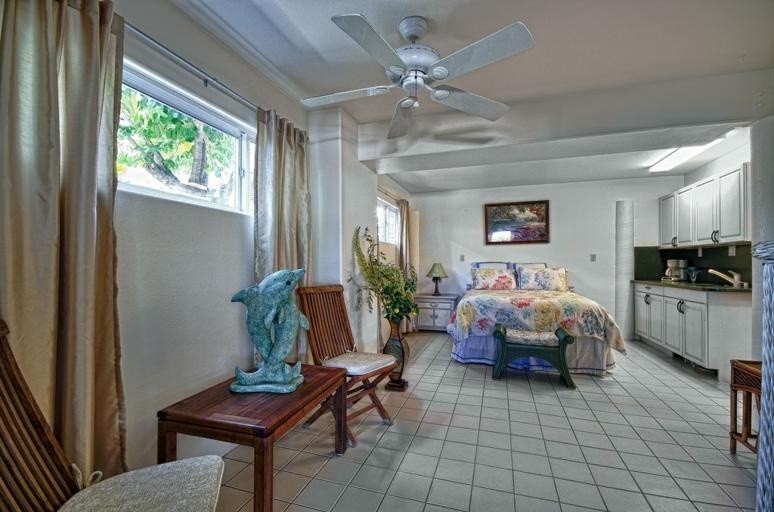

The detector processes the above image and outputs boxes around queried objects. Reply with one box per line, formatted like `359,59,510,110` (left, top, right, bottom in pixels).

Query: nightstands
412,293,459,333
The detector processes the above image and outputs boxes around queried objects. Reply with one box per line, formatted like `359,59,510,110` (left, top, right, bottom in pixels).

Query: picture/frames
481,199,550,244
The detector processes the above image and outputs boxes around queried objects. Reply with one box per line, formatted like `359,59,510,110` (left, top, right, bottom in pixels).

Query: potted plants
346,222,418,392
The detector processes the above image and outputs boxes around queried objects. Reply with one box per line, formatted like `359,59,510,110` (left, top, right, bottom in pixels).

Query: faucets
708,268,741,288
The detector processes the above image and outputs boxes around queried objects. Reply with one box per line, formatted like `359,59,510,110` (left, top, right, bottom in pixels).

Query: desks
156,363,349,511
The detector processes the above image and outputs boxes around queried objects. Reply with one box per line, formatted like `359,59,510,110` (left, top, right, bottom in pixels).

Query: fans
297,15,535,143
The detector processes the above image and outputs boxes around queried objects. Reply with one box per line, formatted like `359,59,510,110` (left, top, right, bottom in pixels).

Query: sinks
698,284,724,288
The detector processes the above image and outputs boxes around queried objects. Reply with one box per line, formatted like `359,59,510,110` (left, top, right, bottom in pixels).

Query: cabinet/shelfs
635,280,665,347
665,287,751,370
695,164,750,245
658,185,692,251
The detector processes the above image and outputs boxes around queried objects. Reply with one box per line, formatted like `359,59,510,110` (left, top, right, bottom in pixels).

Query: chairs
0,319,225,512
296,284,399,444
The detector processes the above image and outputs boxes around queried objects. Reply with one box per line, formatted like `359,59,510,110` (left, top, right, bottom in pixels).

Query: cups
688,267,695,272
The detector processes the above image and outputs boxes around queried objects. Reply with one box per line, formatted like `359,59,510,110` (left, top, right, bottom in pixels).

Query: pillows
470,261,513,270
471,268,516,290
517,269,571,291
510,261,549,286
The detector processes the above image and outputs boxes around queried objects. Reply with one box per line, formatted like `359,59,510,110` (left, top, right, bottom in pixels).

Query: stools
729,357,766,456
490,321,578,389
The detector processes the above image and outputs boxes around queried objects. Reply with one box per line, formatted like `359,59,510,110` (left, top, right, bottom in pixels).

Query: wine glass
689,273,696,284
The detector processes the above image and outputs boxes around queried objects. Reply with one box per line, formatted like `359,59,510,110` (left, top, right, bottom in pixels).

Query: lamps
425,261,448,296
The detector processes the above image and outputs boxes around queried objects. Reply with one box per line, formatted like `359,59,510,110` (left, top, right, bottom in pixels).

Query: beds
447,261,627,375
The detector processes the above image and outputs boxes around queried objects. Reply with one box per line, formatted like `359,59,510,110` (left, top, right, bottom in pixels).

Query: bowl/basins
666,260,687,269
670,270,687,281
